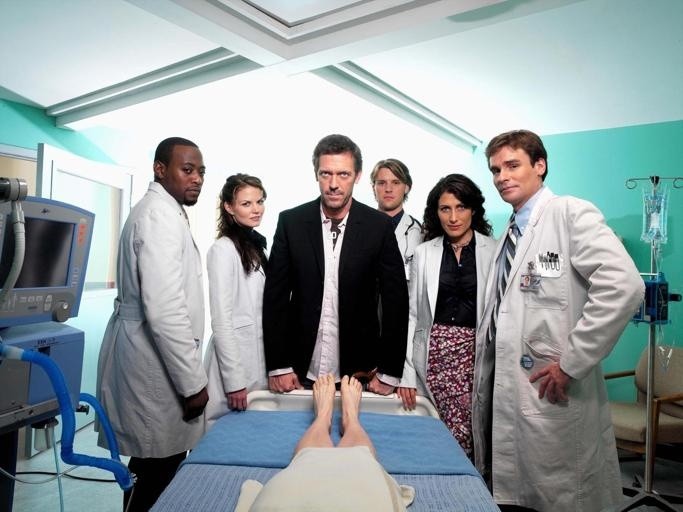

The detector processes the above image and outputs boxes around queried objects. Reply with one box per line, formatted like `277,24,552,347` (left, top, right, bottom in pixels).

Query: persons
369,158,425,284
524,276,529,287
228,371,422,511
200,172,272,421
94,137,210,512
472,130,646,512
261,134,409,397
395,174,503,469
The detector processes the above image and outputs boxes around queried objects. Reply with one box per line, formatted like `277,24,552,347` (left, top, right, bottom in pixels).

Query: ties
486,225,518,347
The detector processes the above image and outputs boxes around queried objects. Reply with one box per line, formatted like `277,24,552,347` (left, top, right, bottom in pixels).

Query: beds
144,390,503,512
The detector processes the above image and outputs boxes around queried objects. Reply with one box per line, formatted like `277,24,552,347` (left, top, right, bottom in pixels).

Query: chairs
604,344,683,512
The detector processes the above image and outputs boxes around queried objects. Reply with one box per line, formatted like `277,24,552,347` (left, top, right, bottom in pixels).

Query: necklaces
448,231,472,252
331,231,337,238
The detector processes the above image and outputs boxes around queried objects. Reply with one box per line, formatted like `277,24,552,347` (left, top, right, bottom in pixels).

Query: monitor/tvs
0,196,95,327
